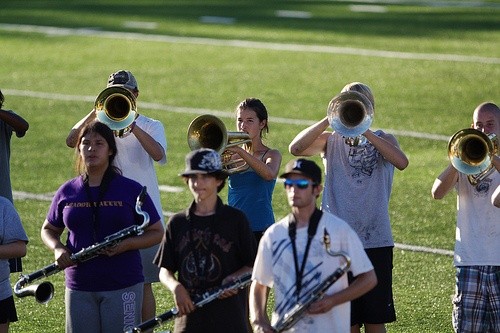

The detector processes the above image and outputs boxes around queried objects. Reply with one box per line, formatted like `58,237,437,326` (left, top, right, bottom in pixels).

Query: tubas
447,127,499,186
94,85,139,139
326,91,375,138
187,112,254,175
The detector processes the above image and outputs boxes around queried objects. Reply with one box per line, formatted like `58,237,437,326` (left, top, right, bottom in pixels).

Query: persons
66,69,168,333
153,147,257,333
40,121,164,333
0,89,30,272
289,82,410,333
431,100,500,333
0,196,29,333
222,97,283,333
249,157,377,333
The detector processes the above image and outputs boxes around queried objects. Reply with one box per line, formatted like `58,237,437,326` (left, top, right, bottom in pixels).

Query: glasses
285,178,317,190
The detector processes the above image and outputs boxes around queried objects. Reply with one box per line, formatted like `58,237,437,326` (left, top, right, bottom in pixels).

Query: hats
178,148,225,174
107,70,136,90
279,158,321,182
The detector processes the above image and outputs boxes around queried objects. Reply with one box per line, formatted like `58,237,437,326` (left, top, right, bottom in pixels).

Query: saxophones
14,185,150,306
127,273,253,333
257,225,353,333
